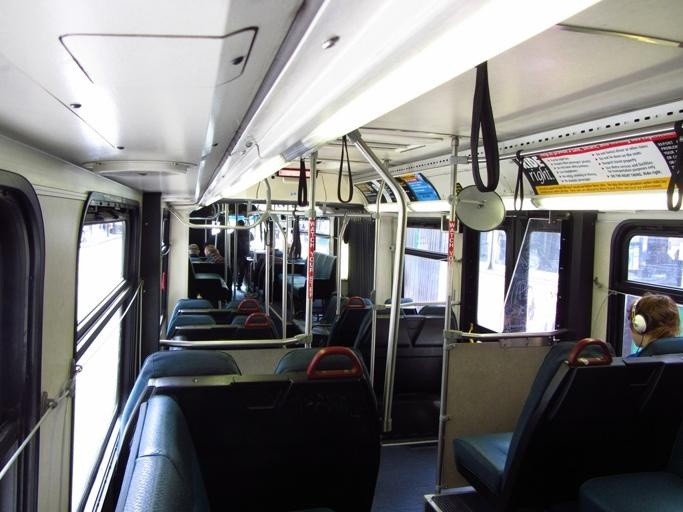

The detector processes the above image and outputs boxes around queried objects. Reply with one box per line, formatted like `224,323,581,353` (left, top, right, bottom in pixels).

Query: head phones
630,296,648,335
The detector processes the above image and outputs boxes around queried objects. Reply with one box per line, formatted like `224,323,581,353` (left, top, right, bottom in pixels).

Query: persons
204,244,220,257
215,221,224,257
230,220,254,290
626,295,683,357
189,244,200,257
